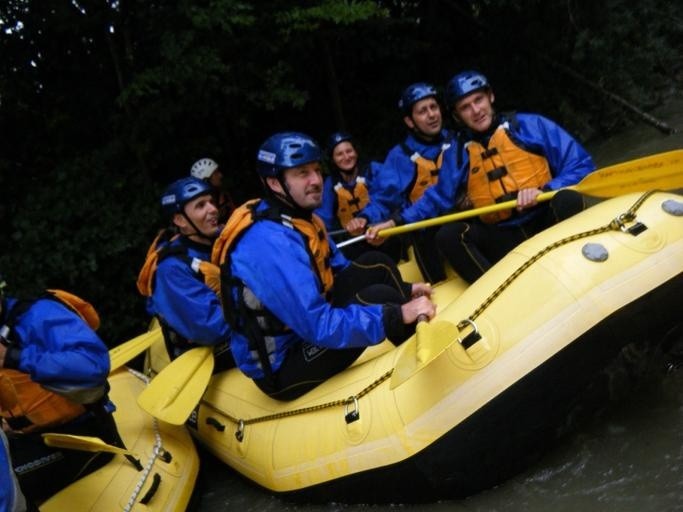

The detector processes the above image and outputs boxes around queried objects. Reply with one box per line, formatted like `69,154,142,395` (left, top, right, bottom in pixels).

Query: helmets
160,178,212,214
190,158,218,180
450,70,490,100
257,132,322,179
399,82,436,108
331,133,350,149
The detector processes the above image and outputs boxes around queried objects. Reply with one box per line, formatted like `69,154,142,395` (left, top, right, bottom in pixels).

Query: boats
1,341,200,507
143,137,681,493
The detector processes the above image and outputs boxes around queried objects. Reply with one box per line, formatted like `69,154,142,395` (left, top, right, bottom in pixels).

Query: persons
309,129,404,267
188,156,240,229
1,269,119,512
209,129,438,404
345,82,459,286
134,176,238,377
367,66,597,286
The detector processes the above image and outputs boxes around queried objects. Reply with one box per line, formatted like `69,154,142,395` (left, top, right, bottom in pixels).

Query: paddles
137,347,214,425
108,328,159,373
375,148,683,235
391,284,459,392
43,433,138,458
